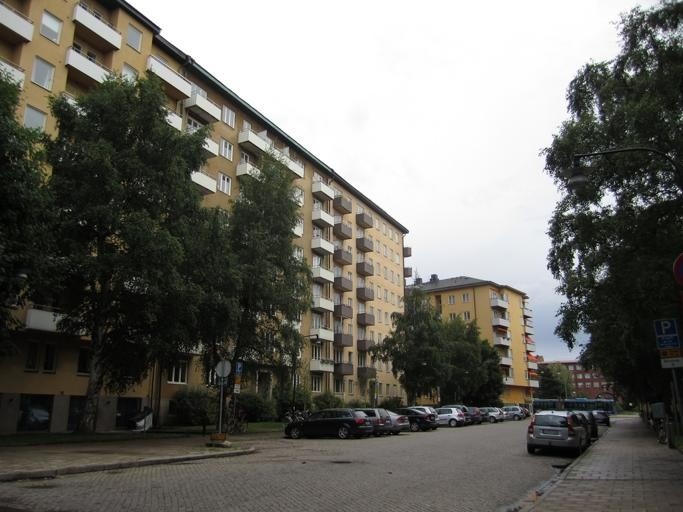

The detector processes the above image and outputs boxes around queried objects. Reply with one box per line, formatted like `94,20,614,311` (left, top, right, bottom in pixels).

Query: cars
526,409,609,455
283,403,529,439
19,405,51,429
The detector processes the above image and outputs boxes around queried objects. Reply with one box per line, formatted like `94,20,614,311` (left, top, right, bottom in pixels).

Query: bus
532,397,615,414
